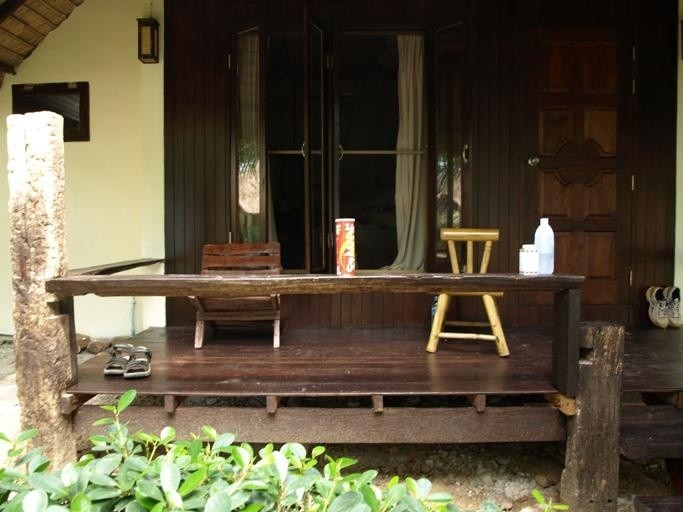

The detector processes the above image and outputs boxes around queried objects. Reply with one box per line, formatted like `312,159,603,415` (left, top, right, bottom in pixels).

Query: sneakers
663,287,681,328
646,286,668,328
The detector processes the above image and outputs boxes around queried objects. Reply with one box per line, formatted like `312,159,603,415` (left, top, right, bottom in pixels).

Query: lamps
136,4,159,64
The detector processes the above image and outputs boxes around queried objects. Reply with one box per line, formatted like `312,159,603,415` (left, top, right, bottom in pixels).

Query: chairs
187,242,281,348
426,228,511,357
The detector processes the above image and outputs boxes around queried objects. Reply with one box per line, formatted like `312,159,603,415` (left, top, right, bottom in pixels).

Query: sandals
123,346,151,378
104,344,133,375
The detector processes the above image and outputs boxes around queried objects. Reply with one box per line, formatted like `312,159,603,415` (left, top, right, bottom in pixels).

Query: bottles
533,217,554,276
516,244,539,275
